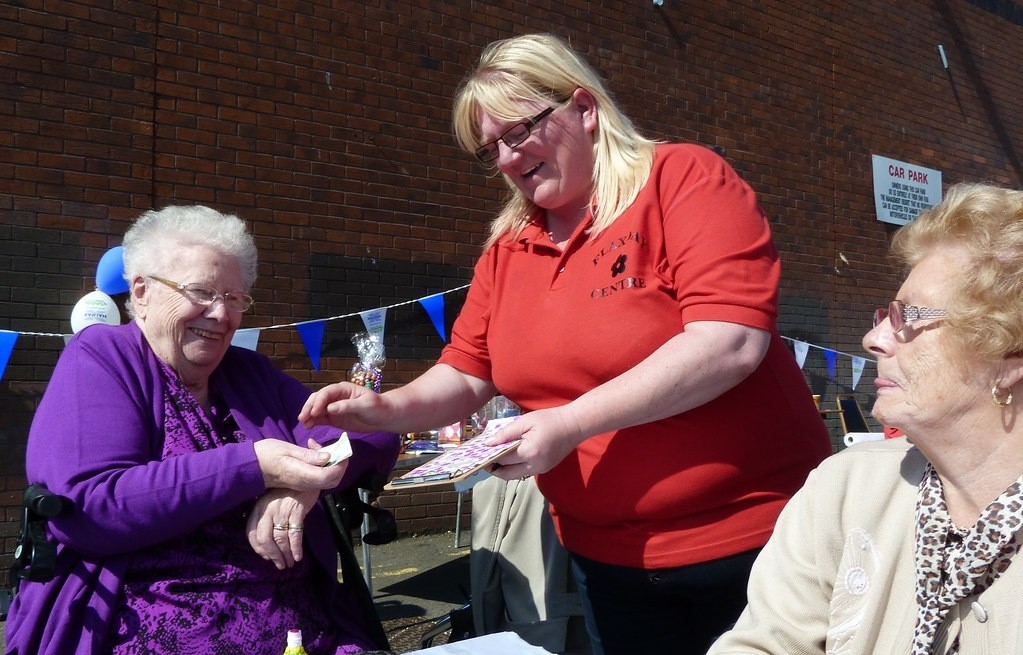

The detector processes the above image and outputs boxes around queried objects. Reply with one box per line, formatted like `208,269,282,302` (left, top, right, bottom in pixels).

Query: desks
355,426,483,592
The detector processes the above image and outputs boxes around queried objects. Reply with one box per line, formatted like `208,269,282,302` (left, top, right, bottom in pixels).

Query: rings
289,525,302,532
273,524,288,530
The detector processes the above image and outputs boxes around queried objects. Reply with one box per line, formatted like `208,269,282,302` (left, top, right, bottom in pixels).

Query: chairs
837,393,872,435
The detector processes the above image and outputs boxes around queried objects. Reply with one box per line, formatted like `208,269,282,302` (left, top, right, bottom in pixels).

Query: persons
297,32,832,655
5,204,400,655
705,181,1023,655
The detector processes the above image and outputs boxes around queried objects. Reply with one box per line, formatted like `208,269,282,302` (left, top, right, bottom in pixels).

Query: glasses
474,97,572,163
872,299,953,335
146,275,255,313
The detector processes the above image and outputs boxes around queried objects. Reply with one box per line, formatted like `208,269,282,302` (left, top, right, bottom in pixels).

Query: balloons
95,245,130,295
70,290,120,334
